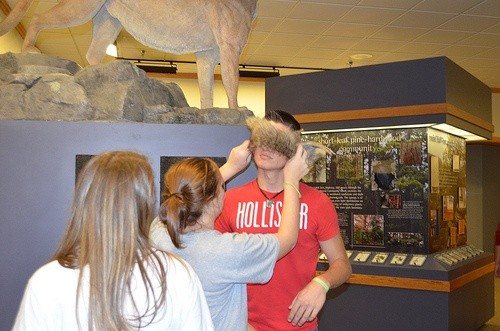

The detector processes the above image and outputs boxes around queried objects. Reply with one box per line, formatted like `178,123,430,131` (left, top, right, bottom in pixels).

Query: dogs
0,0,260,112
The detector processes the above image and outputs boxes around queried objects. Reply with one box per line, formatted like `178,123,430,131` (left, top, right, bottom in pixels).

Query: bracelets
312,277,330,293
284,181,302,199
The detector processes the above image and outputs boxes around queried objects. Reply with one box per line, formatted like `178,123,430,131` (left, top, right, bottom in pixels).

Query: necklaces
256,177,283,207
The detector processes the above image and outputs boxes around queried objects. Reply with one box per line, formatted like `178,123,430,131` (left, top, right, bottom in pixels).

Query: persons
150,140,308,331
214,110,352,331
11,150,216,331
